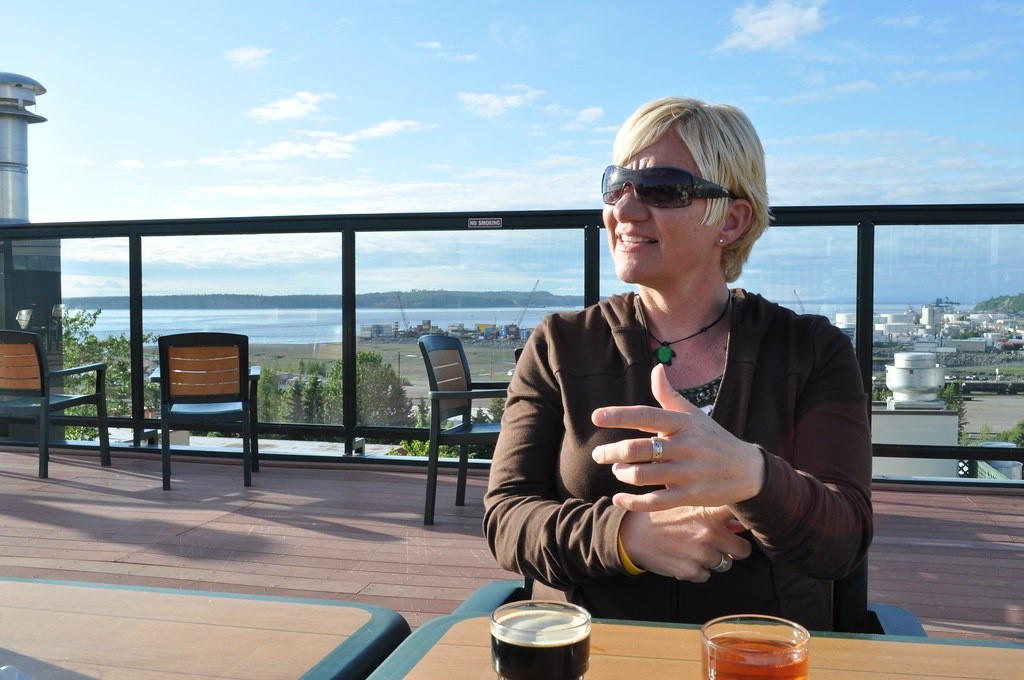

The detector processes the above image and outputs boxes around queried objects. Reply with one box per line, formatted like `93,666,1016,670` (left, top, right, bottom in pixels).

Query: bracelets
619,533,647,575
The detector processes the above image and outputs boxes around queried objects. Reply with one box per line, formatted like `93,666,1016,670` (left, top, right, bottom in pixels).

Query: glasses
599,165,737,209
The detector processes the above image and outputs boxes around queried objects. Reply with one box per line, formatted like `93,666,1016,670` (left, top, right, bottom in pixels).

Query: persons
482,96,875,631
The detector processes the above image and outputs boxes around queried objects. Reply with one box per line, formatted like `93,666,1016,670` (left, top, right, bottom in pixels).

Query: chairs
0,329,112,477
448,550,928,635
149,332,261,487
418,334,512,526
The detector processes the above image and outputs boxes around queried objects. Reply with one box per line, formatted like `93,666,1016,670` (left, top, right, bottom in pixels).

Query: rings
651,437,663,464
710,553,732,573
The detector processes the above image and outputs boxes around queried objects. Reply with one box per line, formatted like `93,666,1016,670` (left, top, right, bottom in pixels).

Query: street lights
16,304,70,355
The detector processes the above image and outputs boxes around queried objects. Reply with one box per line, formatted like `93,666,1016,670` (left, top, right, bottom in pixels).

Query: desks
0,576,413,680
365,610,1024,680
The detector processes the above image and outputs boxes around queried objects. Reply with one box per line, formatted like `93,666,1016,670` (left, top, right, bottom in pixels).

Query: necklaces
649,288,731,367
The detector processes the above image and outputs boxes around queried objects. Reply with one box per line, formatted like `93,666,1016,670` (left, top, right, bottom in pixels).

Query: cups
700,614,810,680
490,599,592,680
